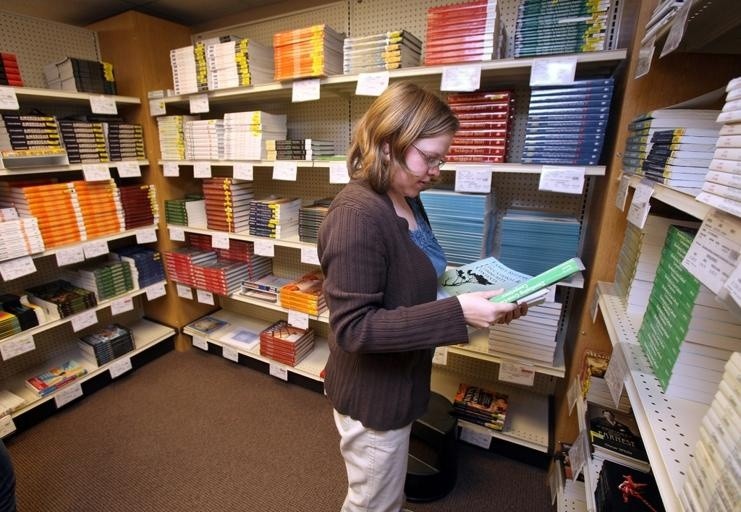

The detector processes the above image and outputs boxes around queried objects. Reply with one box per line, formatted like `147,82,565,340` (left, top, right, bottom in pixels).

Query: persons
311,79,533,509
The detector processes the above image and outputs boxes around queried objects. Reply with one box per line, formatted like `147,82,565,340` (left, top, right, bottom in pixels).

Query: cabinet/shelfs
0,0,741,512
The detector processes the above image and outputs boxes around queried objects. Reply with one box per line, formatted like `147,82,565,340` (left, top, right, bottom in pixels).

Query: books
155,1,616,435
0,49,170,420
555,1,741,511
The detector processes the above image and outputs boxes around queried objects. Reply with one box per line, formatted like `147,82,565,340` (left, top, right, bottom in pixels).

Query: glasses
411,143,448,168
